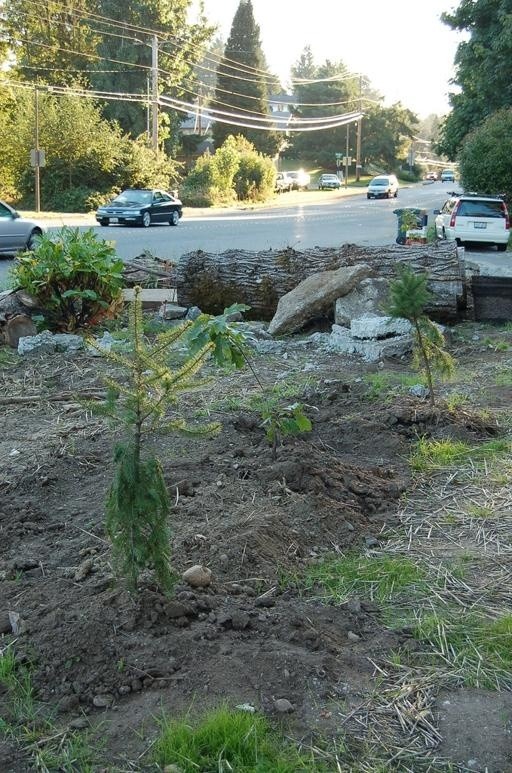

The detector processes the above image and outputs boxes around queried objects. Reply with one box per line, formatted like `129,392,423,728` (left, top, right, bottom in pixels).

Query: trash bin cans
393,208,425,245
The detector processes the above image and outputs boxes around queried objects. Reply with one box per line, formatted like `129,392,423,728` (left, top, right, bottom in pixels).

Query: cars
94,187,184,227
426,171,439,181
0,199,47,258
318,173,341,191
274,170,295,196
288,171,312,192
440,168,456,183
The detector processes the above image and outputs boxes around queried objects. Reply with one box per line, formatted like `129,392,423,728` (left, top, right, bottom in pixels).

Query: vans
366,174,401,200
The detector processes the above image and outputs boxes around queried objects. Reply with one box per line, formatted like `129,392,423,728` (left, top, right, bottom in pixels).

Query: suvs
432,191,511,253
